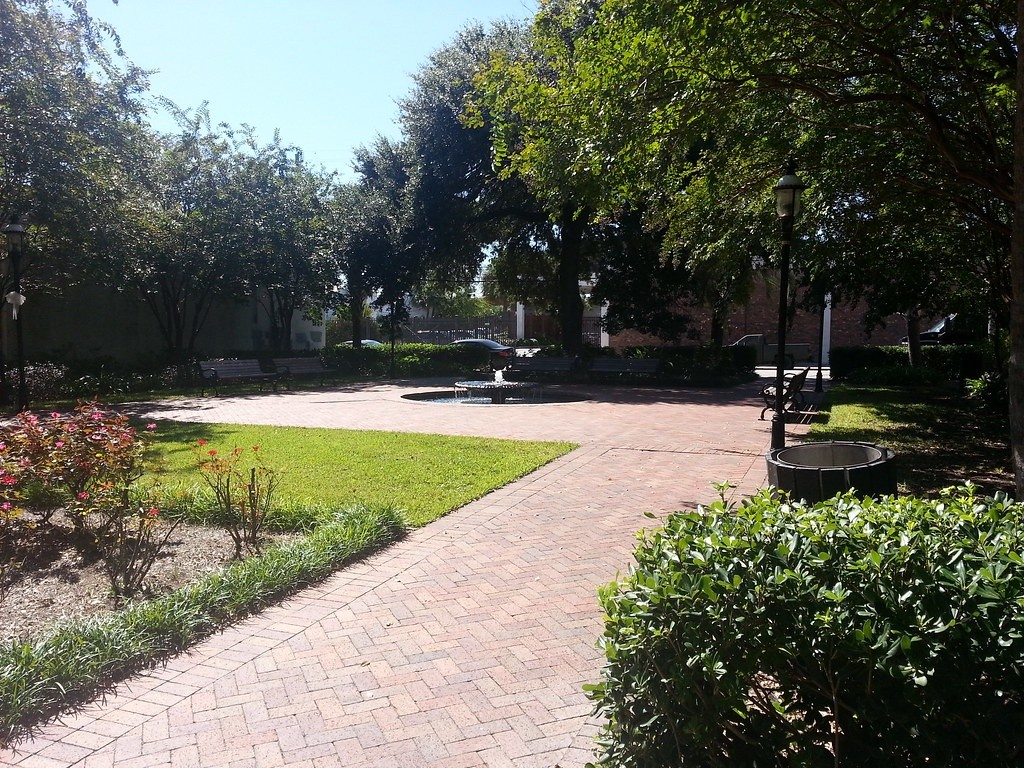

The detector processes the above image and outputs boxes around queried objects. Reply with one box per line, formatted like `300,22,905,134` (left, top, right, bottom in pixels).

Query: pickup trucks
726,335,810,371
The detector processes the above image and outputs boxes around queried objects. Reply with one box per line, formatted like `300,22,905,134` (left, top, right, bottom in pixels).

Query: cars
899,313,960,345
339,339,383,348
447,339,515,371
416,327,493,345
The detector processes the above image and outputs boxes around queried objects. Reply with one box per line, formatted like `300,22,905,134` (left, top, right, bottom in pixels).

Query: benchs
198,359,277,397
587,357,663,388
272,357,337,392
508,357,579,384
758,366,810,420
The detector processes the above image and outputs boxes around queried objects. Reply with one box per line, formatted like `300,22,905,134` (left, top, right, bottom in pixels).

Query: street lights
3,214,33,418
768,165,788,498
